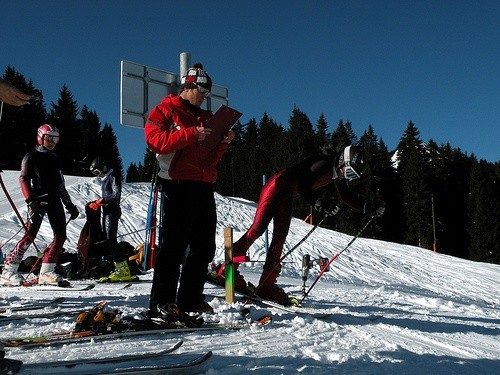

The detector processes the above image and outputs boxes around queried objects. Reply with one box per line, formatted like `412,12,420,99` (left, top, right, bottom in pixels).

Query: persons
90,158,122,261
215,144,388,301
0,124,79,288
142,68,234,319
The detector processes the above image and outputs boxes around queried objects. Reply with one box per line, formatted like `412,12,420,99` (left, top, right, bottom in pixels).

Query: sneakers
39,263,64,285
0,263,23,286
100,261,131,282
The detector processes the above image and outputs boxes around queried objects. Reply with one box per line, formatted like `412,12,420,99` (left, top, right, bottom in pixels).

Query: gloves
65,202,80,220
315,193,343,217
89,197,106,210
25,196,44,224
364,198,386,217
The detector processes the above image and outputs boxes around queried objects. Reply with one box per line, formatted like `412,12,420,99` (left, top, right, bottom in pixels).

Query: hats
179,63,212,92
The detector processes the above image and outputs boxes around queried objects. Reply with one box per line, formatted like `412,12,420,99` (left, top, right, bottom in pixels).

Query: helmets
37,124,60,144
337,146,368,176
90,159,108,175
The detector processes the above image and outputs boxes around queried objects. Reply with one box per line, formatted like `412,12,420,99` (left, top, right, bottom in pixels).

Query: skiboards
206,275,332,319
0,282,137,290
0,338,214,375
0,314,272,347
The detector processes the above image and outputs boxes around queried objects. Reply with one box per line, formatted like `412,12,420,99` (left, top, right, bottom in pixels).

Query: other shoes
151,302,181,321
177,301,214,314
255,285,292,307
216,263,247,288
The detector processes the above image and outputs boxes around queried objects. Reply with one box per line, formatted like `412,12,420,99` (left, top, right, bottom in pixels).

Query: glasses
92,169,101,175
344,145,360,181
44,135,59,143
185,80,211,98
